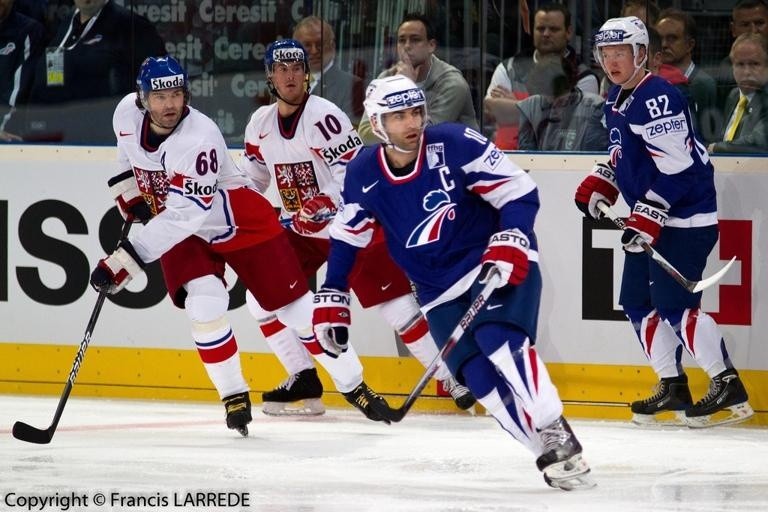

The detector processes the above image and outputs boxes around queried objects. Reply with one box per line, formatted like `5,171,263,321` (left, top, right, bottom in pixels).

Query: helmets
136,56,191,110
265,39,309,81
594,16,649,67
363,75,427,142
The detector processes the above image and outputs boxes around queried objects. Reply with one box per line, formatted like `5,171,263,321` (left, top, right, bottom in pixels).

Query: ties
725,94,748,140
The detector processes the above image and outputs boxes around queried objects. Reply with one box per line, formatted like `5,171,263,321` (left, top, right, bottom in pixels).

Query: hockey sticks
597,202,736,292
362,274,497,421
12,213,139,443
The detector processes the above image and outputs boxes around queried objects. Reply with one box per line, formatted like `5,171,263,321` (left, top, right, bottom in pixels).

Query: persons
312,75,597,491
574,16,754,427
89,56,391,434
0,1,766,158
234,39,478,418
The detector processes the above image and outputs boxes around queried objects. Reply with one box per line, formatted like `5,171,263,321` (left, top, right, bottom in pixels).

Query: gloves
107,168,148,223
312,290,350,358
621,199,670,254
576,165,621,223
289,192,338,236
91,240,145,295
478,228,531,296
